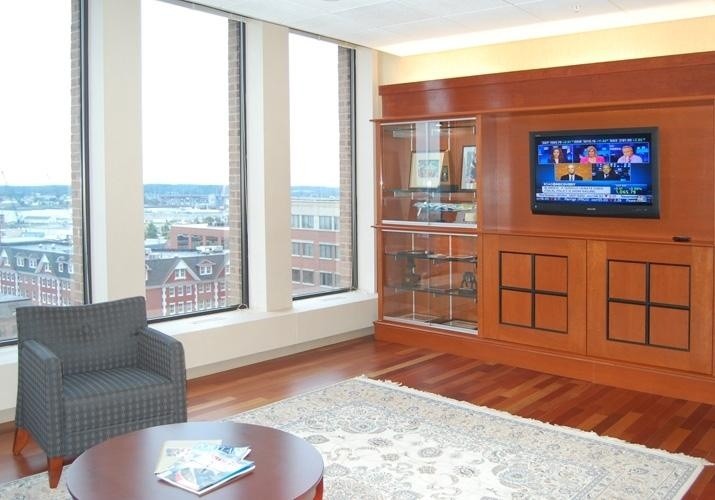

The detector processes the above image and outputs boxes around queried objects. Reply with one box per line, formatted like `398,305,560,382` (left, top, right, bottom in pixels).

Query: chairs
11,295,189,488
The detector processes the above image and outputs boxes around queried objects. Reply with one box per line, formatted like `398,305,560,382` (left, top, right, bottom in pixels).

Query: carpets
2,373,714,499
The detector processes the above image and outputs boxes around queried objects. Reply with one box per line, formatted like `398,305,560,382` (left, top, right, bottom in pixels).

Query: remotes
671,236,692,241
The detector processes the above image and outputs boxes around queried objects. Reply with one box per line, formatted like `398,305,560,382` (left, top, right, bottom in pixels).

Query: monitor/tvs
528,126,660,220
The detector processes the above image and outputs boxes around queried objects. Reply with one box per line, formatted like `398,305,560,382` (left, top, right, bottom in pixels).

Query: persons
594,164,619,180
560,164,583,180
548,148,569,163
616,145,642,162
579,146,605,162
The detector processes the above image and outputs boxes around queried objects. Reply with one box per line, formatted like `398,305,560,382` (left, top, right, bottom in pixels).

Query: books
220,446,251,460
151,446,256,494
153,439,223,474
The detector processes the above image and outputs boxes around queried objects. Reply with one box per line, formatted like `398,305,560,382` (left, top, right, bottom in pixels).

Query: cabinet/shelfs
369,110,496,364
